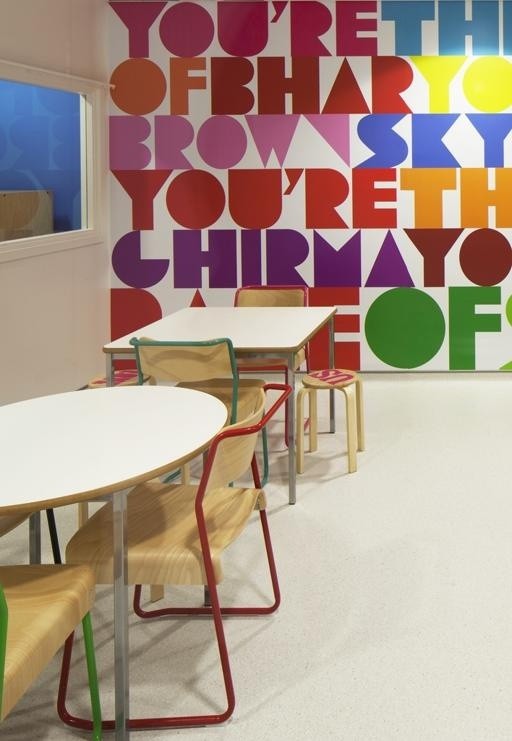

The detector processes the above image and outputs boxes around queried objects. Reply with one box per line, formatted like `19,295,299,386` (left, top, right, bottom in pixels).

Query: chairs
0,562,99,741
126,336,270,497
231,287,310,448
44,381,291,733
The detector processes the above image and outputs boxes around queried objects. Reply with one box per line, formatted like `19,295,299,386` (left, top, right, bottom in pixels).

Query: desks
1,385,230,740
102,305,338,508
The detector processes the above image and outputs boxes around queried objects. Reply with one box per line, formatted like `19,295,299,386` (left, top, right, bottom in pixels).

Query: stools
295,369,365,477
